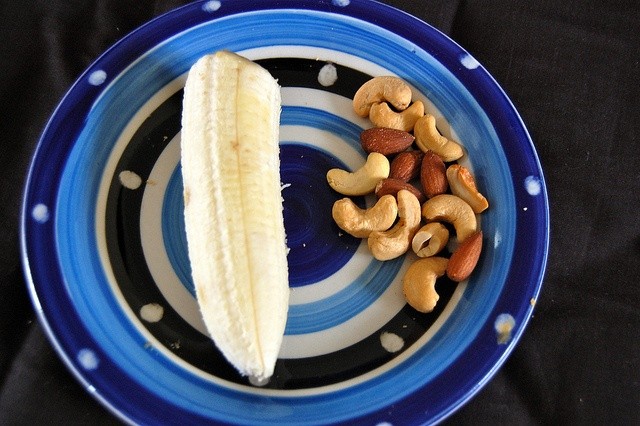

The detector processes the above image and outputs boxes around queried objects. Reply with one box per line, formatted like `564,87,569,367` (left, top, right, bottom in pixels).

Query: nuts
326,75,489,315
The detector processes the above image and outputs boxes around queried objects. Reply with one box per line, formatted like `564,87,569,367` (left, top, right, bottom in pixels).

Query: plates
22,3,551,426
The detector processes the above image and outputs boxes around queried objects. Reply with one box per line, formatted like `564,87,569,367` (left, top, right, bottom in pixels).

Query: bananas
180,51,290,386
